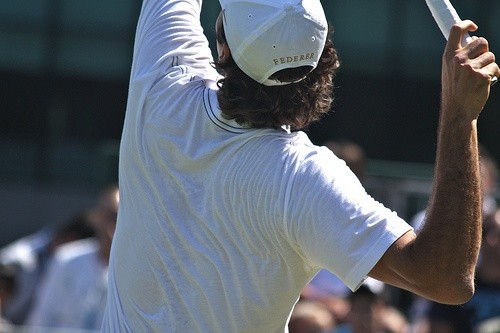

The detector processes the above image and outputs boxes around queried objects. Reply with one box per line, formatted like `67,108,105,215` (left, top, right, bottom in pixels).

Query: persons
0,138,500,333
98,0,500,332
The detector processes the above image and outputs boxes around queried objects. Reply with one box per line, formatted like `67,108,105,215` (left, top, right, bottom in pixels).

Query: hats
218,0,329,87
